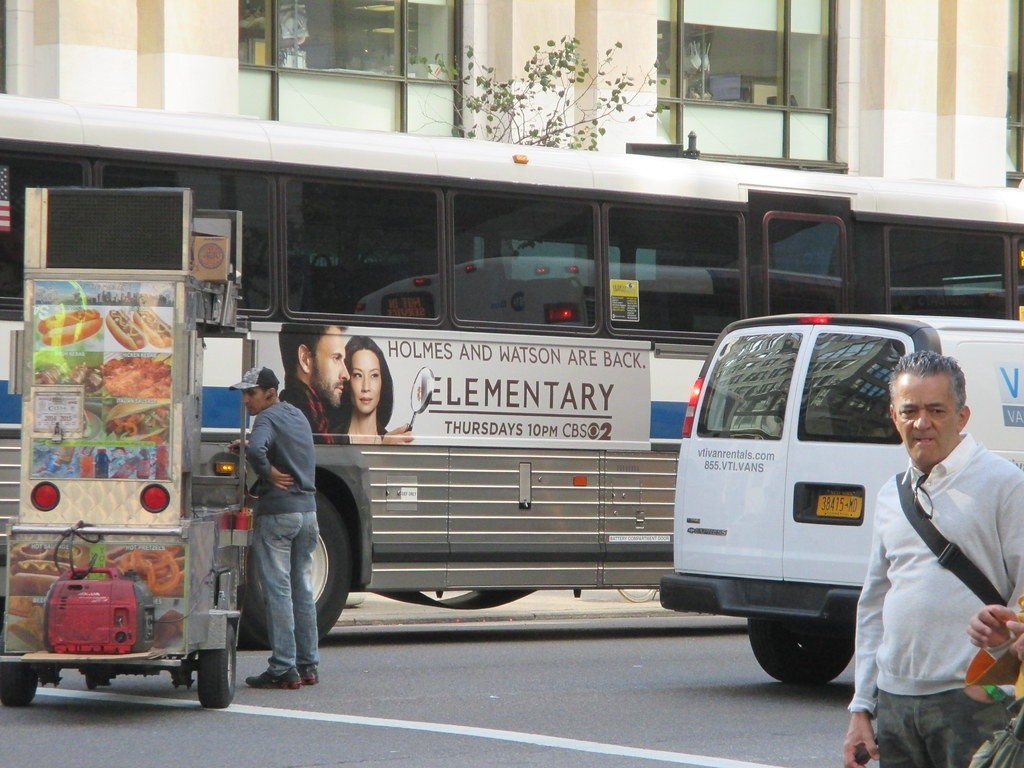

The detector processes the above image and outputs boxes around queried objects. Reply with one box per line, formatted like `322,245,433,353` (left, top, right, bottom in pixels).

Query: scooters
0,185,259,708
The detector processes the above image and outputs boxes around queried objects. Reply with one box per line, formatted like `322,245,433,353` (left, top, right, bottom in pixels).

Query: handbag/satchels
966,704,1024,767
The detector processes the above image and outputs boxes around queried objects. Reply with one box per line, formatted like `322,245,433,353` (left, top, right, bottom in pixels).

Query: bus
0,94,1024,610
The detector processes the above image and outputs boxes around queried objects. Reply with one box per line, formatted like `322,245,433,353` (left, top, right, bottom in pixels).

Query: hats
228,366,280,391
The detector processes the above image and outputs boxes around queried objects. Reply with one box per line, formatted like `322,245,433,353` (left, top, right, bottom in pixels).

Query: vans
656,310,1024,686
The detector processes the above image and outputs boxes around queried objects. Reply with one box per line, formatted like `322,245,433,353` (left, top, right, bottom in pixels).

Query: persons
965,593,1023,700
842,350,1023,768
279,323,416,445
229,366,320,688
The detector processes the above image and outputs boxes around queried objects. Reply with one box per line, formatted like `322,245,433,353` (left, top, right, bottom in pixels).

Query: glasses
913,473,934,521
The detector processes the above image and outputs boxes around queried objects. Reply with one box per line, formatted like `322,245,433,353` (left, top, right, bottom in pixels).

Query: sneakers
297,664,320,686
246,666,302,690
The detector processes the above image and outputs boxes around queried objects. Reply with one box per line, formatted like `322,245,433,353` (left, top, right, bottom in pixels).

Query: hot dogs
38,309,103,346
106,307,174,351
9,542,92,596
